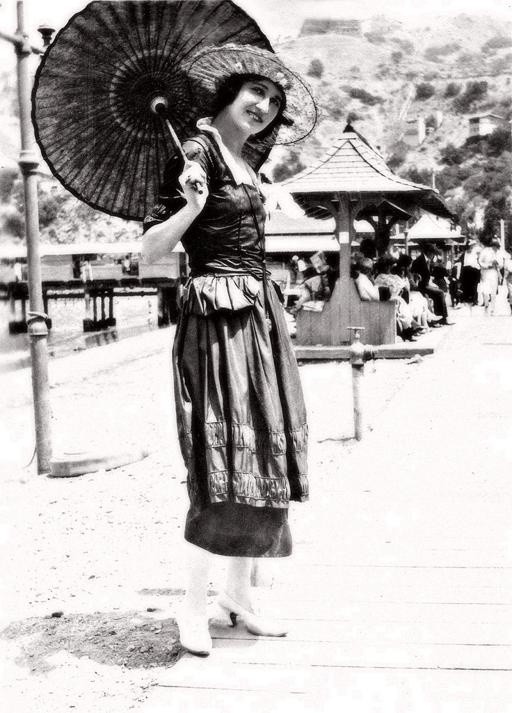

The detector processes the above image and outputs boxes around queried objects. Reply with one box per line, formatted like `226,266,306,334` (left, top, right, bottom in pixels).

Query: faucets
347,324,378,368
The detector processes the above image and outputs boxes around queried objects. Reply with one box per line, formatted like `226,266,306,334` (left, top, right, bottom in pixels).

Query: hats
186,43,319,149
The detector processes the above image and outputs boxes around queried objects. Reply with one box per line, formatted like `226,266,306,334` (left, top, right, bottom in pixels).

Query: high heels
218,591,287,637
173,600,212,655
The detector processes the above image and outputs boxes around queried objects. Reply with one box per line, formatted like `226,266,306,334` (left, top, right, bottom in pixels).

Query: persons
139,76,310,636
287,239,512,335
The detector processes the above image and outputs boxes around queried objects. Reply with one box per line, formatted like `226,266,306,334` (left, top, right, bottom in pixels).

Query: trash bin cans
156,283,181,328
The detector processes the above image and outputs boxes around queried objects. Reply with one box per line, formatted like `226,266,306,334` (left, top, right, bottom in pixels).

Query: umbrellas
31,0,288,221
